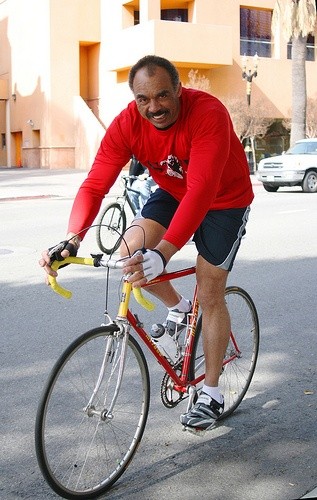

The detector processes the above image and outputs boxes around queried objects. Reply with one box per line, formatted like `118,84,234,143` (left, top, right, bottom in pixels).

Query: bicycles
96,175,159,254
34,223,260,500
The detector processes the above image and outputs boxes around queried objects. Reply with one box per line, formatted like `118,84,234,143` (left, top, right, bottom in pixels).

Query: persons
39,55,254,427
129,159,156,211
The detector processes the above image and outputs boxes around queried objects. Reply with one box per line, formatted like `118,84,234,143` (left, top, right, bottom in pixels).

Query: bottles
150,323,182,366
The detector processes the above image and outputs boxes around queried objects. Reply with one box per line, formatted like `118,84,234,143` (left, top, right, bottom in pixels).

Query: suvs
257,136,317,193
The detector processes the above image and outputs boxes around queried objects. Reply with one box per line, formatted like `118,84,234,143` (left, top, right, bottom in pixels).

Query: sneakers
150,299,193,344
180,391,225,426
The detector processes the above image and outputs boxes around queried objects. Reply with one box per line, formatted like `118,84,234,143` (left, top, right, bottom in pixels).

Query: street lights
242,51,260,107
